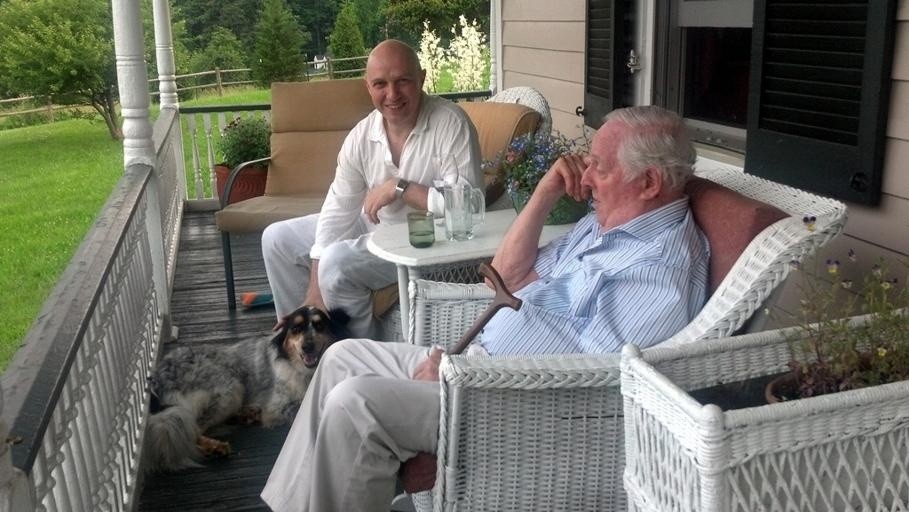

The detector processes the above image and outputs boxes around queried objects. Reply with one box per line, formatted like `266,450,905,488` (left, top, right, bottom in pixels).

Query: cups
407,184,488,249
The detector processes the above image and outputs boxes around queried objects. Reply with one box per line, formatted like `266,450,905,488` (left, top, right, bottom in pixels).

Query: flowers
219,116,270,165
761,217,909,369
480,126,593,192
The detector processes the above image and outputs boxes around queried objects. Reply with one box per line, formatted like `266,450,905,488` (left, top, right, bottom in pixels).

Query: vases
510,192,590,226
761,370,909,404
215,165,268,208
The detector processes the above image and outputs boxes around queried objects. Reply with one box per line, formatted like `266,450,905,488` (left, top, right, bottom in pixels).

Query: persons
261,38,486,340
259,106,711,512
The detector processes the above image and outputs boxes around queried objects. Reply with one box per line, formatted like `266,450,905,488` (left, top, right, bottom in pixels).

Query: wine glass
429,152,464,227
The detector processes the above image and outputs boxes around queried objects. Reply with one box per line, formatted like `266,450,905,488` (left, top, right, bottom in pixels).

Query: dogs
129,305,356,479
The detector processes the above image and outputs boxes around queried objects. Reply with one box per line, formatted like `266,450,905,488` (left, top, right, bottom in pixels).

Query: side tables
366,206,575,342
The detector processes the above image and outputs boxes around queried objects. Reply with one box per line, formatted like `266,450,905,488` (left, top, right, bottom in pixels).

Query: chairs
373,87,551,350
214,78,376,312
398,156,849,512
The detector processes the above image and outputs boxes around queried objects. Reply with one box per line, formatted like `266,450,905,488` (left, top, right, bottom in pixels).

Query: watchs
395,179,409,201
